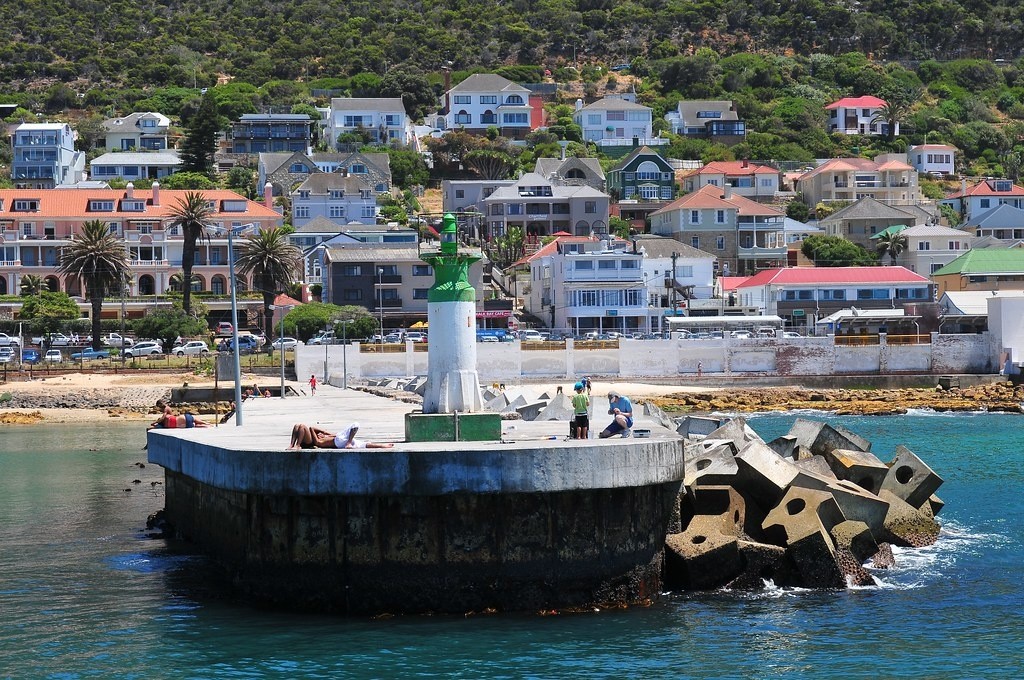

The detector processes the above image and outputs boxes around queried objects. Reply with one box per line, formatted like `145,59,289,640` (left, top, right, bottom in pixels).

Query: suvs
213,321,234,337
121,341,164,358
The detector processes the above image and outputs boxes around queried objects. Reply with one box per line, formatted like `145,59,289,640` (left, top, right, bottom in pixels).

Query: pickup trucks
92,333,135,347
30,332,74,346
71,347,109,361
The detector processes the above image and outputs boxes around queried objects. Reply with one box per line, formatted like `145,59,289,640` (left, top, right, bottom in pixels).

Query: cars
22,350,40,364
172,340,211,357
45,349,64,365
240,330,429,345
215,337,256,352
0,333,25,346
482,329,801,341
0,347,17,364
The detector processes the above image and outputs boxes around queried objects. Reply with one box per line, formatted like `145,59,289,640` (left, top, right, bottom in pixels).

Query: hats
574,382,584,390
608,391,617,404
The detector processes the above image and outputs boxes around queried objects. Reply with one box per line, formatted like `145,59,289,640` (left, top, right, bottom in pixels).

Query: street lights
334,318,355,391
377,268,384,344
269,304,297,400
202,223,255,427
720,264,728,315
318,329,335,385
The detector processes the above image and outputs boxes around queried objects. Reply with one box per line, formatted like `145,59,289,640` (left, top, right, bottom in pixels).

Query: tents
410,321,428,332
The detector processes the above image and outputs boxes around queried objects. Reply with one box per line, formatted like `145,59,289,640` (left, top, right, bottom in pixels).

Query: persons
210,332,215,345
242,383,271,398
698,363,702,376
220,338,227,351
557,386,562,396
141,400,173,451
286,424,394,449
228,400,235,413
572,377,592,440
67,331,93,347
599,391,634,438
494,383,505,389
158,414,211,427
308,375,319,396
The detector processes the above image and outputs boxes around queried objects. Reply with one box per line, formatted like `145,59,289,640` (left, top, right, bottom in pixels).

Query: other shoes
621,430,631,438
